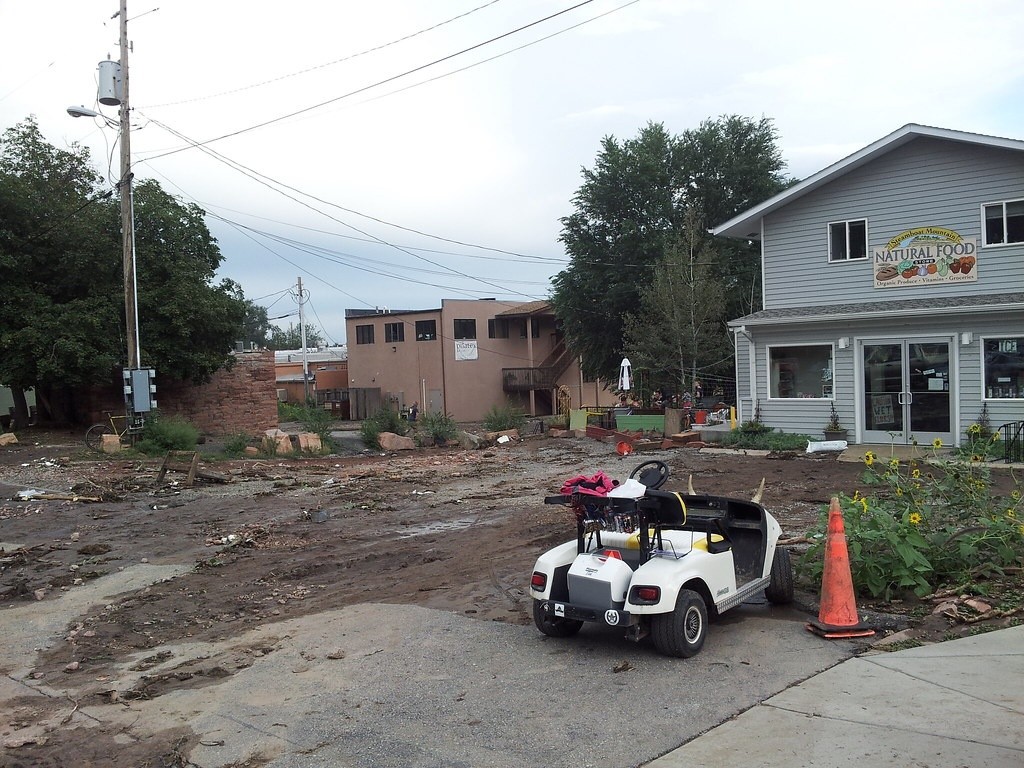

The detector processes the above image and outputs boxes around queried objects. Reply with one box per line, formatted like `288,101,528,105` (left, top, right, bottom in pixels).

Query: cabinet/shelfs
772,358,798,397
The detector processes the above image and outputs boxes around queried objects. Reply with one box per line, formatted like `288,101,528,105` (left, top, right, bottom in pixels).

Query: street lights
66,104,139,367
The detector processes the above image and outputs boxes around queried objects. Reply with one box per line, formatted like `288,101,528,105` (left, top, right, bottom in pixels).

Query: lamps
740,326,754,343
962,331,974,344
391,347,396,352
838,337,850,349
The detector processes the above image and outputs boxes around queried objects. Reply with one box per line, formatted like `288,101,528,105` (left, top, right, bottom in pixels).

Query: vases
823,430,847,441
966,431,992,439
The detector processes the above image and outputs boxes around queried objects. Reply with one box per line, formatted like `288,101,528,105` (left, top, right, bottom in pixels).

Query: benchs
590,489,734,553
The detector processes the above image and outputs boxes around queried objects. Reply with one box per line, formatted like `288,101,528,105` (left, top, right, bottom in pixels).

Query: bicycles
85,410,132,453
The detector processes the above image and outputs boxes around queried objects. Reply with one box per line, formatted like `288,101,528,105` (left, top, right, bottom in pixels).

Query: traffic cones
804,497,877,639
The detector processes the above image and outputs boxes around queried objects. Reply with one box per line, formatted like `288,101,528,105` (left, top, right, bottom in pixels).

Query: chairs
707,409,728,424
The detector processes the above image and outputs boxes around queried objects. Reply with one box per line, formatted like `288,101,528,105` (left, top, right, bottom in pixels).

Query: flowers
823,401,848,430
973,402,994,432
